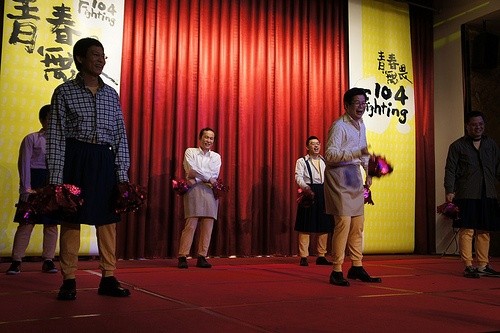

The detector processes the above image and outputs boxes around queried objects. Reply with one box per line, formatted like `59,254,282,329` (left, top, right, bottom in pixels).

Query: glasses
350,100,368,107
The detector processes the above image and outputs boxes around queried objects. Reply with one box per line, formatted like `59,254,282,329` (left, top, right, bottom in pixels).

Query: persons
441,115,499,278
324,86,382,286
46,38,130,301
175,127,222,268
293,135,333,266
8,104,61,273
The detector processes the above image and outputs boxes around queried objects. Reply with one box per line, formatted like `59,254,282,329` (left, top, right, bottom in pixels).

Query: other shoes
347,266,382,282
41,260,57,273
463,265,500,278
330,272,350,287
58,279,77,300
300,257,309,267
316,257,333,265
98,276,131,296
197,257,211,268
7,264,20,273
177,257,188,268
